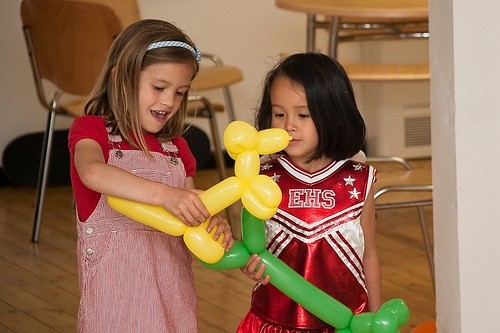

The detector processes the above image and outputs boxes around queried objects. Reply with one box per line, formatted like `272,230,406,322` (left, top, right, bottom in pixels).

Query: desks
275,0,429,60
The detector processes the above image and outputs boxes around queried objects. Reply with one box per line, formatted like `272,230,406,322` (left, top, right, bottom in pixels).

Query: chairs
19,0,238,242
366,155,435,286
78,0,243,125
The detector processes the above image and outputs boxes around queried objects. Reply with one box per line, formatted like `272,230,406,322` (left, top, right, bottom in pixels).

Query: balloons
200,206,409,333
106,120,293,264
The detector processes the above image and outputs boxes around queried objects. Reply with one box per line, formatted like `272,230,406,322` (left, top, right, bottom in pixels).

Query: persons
65,18,233,333
233,52,382,333
